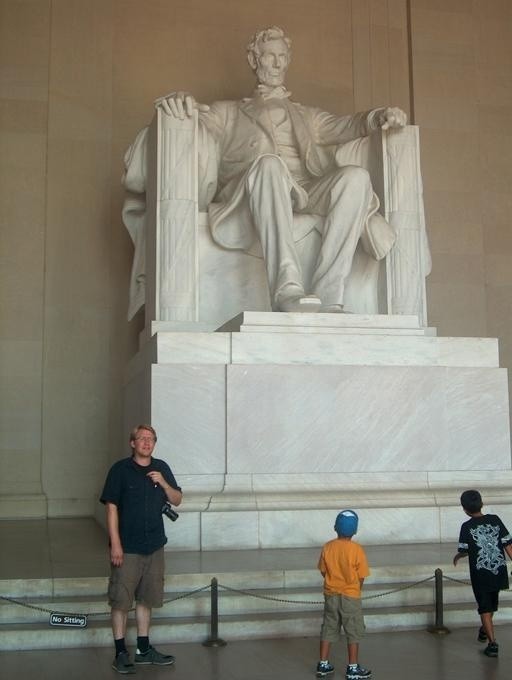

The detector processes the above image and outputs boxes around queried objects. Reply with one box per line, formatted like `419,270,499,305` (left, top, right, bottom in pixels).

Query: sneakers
112,650,136,673
317,660,334,677
478,627,498,657
346,664,371,680
136,645,175,665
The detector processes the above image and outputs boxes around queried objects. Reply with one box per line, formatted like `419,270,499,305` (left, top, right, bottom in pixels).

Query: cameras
160,504,179,522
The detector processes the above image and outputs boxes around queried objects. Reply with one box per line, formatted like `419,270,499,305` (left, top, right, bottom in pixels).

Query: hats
336,510,357,535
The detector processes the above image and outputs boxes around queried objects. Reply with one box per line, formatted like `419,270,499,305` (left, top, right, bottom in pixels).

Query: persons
100,423,183,674
454,490,512,656
316,510,371,680
154,24,408,313
